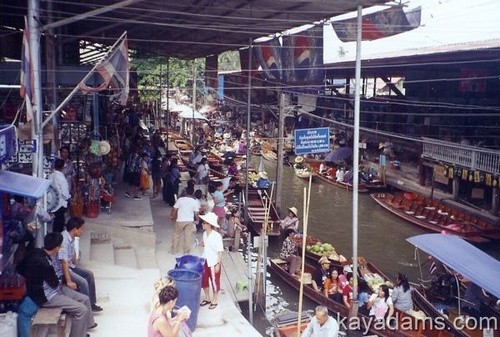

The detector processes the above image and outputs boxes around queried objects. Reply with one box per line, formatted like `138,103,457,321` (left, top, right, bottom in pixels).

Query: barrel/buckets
166,269,202,332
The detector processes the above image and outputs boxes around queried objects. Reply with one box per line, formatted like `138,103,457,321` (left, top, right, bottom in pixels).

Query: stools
33,306,67,337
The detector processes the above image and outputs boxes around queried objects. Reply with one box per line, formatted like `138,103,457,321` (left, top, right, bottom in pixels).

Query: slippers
209,303,218,309
200,300,211,306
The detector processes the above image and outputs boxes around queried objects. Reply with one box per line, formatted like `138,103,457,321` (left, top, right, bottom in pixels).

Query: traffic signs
293,126,330,224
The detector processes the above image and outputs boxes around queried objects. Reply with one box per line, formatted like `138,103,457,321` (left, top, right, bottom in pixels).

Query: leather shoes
88,323,97,328
92,304,102,311
86,334,90,337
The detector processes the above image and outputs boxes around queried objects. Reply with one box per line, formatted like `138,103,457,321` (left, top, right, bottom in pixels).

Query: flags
79,31,129,105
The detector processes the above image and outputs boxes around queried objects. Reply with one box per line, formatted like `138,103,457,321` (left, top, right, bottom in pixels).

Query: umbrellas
325,147,353,168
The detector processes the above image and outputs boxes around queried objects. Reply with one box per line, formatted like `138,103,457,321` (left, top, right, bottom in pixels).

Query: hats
318,257,330,265
295,156,303,163
202,149,207,152
258,171,268,180
90,164,102,178
199,212,220,228
289,207,298,216
241,155,246,159
78,142,88,154
99,141,110,155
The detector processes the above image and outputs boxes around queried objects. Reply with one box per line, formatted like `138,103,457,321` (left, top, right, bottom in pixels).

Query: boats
259,149,279,162
304,161,388,194
298,246,347,277
205,151,227,179
238,186,283,238
274,314,311,337
406,232,500,336
164,127,201,171
368,191,500,247
292,162,314,179
268,257,456,337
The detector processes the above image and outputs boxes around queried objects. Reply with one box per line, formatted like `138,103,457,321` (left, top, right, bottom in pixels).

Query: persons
301,305,340,337
276,206,299,231
168,187,200,255
198,211,224,310
294,156,378,185
342,272,354,309
367,284,394,325
214,131,247,154
391,272,413,313
124,126,162,201
213,181,235,234
150,276,193,337
220,177,239,196
50,145,77,226
324,266,343,303
256,171,272,188
357,279,369,315
50,216,102,311
279,229,302,277
148,286,192,336
47,159,72,233
337,264,353,294
311,256,331,293
180,145,215,201
160,154,181,207
25,232,97,337
220,146,239,176
227,209,249,237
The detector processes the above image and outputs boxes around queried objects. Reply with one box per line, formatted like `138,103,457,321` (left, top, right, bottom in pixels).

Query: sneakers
125,192,130,198
134,197,143,200
